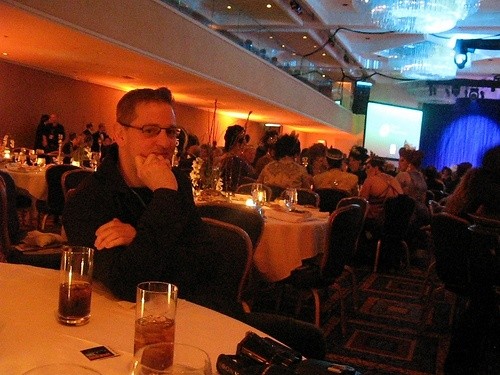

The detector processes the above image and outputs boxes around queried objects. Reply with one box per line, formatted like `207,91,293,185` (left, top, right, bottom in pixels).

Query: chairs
0,164,500,361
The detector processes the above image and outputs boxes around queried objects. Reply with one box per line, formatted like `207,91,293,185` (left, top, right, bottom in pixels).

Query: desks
0,262,309,375
194,192,334,283
0,158,55,228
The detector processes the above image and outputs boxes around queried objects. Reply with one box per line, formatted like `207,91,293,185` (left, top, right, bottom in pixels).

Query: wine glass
30,154,38,167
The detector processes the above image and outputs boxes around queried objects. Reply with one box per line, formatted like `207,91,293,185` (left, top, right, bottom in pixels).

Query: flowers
189,157,223,203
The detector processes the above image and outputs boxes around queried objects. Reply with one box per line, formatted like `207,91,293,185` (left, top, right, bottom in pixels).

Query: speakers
351,82,370,115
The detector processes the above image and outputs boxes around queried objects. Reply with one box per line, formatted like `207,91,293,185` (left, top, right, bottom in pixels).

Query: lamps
371,40,472,80
392,80,456,105
352,0,481,34
465,88,484,106
453,39,500,69
452,85,460,97
491,87,495,92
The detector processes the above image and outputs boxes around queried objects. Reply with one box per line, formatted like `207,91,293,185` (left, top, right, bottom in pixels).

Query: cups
22,363,102,375
129,342,213,375
56,246,94,326
251,183,268,213
37,158,46,167
284,187,301,212
133,281,178,375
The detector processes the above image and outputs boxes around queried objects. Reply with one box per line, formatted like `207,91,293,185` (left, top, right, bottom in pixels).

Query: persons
212,125,480,249
60,87,329,361
33,113,114,169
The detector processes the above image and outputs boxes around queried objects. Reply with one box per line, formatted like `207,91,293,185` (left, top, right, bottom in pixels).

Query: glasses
119,122,181,138
